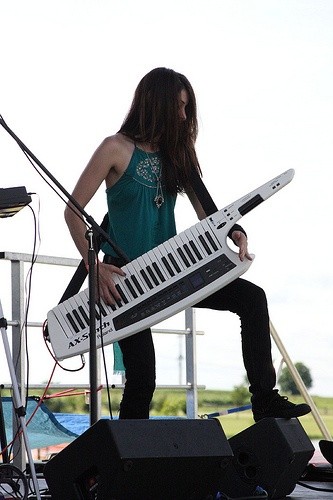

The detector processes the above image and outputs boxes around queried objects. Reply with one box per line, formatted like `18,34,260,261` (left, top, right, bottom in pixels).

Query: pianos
47,168,300,362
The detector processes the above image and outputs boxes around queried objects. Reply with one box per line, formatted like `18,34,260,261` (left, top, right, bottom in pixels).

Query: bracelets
226,224,247,246
85,258,99,271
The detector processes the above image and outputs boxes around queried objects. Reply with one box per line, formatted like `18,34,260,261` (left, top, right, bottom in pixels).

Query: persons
64,66,312,422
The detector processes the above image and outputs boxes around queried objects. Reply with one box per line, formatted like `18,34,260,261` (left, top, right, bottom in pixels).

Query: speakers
226,417,315,498
42,418,234,500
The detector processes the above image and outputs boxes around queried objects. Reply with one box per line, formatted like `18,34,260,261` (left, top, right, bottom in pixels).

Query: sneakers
252,392,311,422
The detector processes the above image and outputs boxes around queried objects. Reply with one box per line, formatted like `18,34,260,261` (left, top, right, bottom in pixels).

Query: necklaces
144,147,165,208
